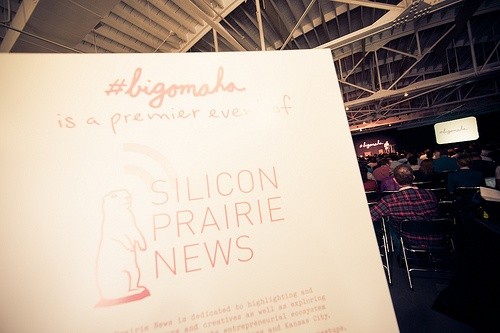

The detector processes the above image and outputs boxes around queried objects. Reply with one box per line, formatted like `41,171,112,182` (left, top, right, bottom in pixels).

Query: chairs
364,164,499,299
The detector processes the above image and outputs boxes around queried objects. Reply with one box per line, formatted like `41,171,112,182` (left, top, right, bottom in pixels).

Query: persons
357,147,500,270
